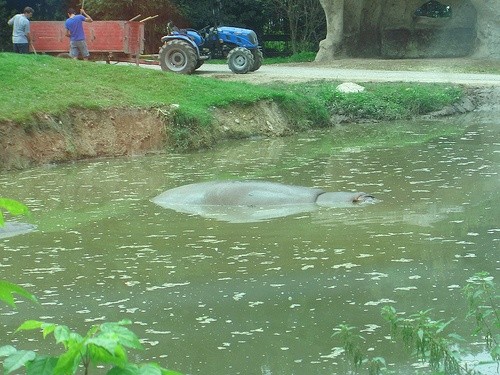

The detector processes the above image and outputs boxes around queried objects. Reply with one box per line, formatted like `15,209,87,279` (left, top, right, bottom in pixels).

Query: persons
65,7,93,61
7,6,34,54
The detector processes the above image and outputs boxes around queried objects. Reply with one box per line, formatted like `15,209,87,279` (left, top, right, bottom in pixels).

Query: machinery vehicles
27,20,264,74
